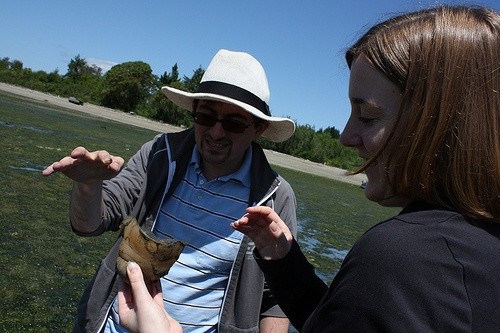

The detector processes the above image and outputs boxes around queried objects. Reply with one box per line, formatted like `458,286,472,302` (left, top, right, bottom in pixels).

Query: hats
161,49,296,142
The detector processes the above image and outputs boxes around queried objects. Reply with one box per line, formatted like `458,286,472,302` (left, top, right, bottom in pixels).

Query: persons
118,4,500,333
41,49,298,333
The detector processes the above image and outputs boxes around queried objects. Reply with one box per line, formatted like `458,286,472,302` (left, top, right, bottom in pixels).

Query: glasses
192,101,252,134
